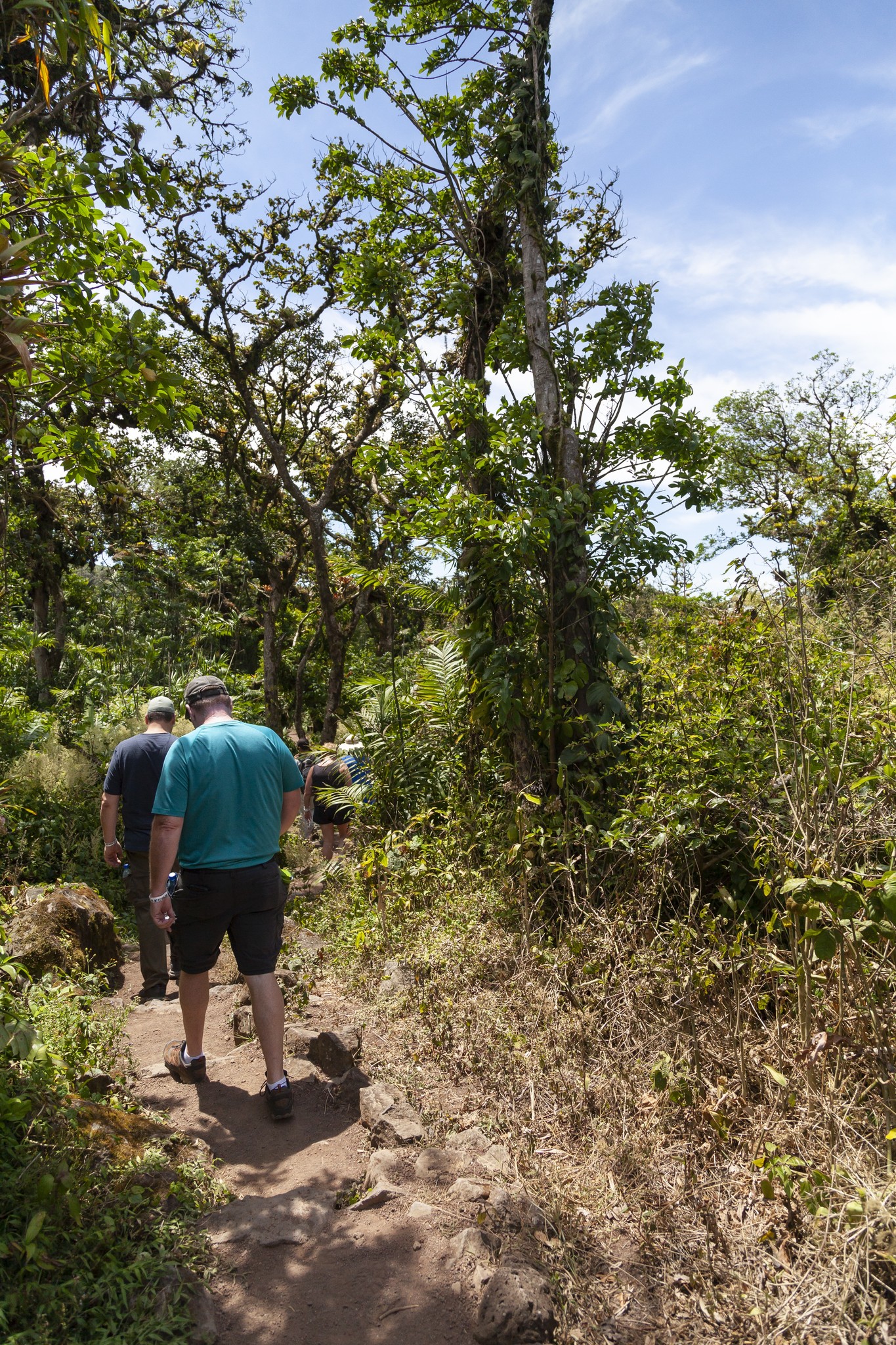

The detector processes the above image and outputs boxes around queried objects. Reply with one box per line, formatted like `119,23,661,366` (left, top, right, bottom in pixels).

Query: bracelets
303,806,310,813
149,891,168,903
104,838,117,847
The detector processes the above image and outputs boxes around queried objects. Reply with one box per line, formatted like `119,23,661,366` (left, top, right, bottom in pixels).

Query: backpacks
297,753,317,795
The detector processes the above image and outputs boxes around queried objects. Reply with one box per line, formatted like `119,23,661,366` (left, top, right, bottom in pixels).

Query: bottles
122,864,132,878
167,872,177,895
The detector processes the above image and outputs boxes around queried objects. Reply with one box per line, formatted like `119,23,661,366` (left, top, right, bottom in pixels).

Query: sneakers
163,1039,207,1082
260,1069,294,1118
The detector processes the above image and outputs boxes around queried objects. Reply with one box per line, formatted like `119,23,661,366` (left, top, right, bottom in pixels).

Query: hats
184,676,230,720
338,735,364,751
147,695,175,714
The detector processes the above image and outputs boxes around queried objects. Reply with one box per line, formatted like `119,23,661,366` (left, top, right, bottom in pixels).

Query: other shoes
168,958,182,979
138,985,167,1000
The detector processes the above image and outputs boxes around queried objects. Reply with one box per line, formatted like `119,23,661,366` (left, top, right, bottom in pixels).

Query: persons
303,742,356,880
148,675,305,1122
100,695,183,1003
293,734,381,848
